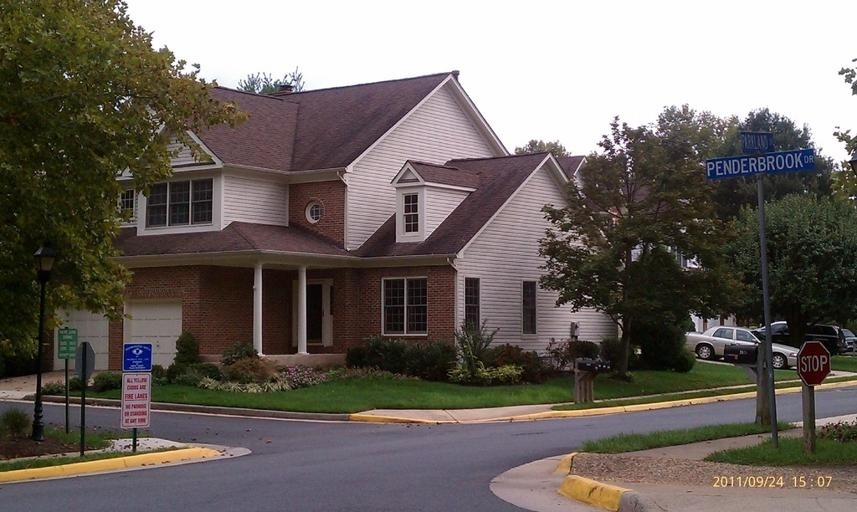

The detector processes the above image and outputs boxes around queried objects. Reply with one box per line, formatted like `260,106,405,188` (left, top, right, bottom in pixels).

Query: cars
838,328,856,353
684,326,801,369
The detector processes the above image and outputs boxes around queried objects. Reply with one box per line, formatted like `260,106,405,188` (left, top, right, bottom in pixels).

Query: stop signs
796,340,834,386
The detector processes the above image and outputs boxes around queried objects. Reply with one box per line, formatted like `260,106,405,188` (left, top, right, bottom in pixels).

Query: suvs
751,322,845,362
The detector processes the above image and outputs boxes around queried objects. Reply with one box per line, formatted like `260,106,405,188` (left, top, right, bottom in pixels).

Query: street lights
25,239,58,441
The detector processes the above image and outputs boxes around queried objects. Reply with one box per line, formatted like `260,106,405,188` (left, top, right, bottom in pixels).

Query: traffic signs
704,147,817,180
735,129,778,154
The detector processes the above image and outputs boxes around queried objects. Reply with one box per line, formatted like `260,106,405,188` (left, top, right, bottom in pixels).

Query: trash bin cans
574,360,595,403
